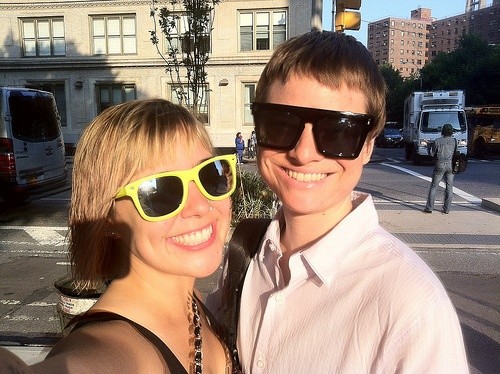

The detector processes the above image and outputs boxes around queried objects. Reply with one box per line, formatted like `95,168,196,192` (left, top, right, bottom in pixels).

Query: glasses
248,101,375,160
114,153,238,223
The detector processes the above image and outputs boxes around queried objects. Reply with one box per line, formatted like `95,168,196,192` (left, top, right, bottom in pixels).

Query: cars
376,128,404,148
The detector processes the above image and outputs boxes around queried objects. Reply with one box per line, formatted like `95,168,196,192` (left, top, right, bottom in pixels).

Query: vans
0,87,67,203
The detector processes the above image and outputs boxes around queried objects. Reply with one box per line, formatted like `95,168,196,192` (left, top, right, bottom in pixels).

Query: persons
220,29,468,374
248,130,256,158
235,133,244,160
0,99,236,374
425,123,459,214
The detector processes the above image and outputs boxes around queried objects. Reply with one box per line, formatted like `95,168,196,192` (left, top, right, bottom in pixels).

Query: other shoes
444,210,449,214
423,209,432,214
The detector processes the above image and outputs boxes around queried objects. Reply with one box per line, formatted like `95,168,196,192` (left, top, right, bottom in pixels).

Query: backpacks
452,137,469,175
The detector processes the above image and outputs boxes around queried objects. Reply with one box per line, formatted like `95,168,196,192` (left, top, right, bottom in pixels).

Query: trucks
403,89,469,164
464,105,500,158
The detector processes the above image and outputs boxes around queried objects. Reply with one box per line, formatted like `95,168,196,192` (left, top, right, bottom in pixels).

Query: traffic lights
335,0,362,34
